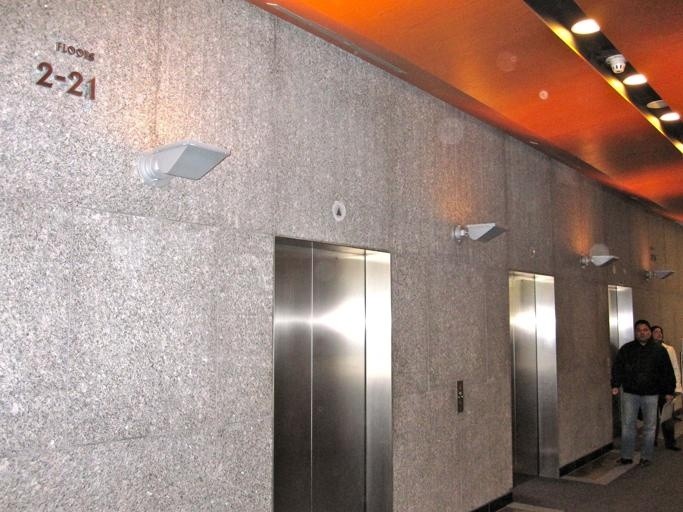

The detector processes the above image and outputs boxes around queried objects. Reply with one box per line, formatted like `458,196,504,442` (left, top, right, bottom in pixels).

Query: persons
649,324,683,452
610,319,677,467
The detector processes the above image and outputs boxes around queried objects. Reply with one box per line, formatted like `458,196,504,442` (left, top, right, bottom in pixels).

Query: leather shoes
620,457,633,464
665,444,680,451
640,458,649,467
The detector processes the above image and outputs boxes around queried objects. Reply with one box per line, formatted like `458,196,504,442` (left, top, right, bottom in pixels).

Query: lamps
136,142,229,186
644,268,675,281
454,221,505,244
578,252,620,269
